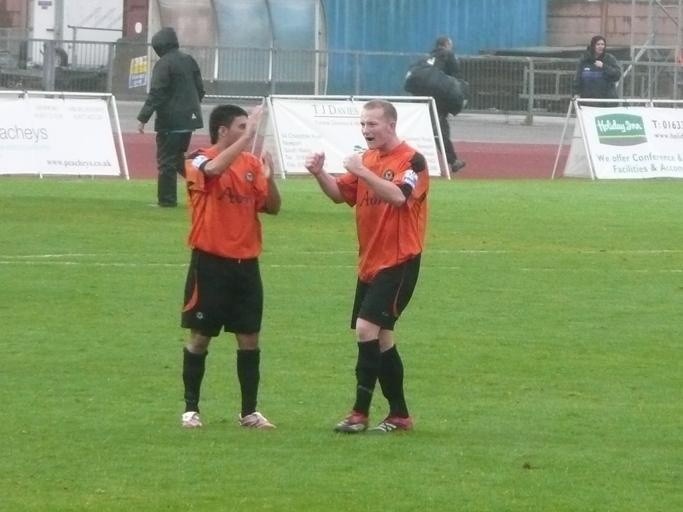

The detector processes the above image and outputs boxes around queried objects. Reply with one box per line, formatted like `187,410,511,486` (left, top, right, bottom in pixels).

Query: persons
134,27,204,208
304,97,429,433
424,36,469,172
572,36,623,108
177,99,281,431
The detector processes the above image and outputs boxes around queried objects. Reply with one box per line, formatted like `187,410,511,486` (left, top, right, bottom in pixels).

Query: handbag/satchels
402,49,470,115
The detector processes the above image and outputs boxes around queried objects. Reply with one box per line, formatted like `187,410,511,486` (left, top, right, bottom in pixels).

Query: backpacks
580,62,620,107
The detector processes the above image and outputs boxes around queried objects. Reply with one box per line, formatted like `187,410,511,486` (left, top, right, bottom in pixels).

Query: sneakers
449,159,468,174
237,411,277,433
369,413,414,434
334,409,371,434
180,409,202,428
